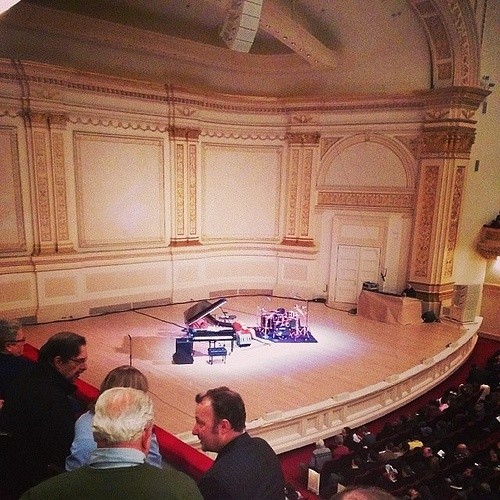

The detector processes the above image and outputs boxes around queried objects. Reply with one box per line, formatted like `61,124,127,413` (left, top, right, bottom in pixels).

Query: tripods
290,314,310,341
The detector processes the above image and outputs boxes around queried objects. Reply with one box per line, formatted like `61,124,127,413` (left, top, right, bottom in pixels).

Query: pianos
183,298,236,360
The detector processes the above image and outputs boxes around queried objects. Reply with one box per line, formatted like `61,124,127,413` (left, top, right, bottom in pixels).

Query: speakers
236,334,252,347
176,338,195,364
422,311,438,322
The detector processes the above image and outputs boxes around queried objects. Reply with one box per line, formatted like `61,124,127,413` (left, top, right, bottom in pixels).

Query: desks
356,289,423,326
219,314,237,322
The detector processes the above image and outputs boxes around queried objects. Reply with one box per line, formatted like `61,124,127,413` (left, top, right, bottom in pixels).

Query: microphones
128,335,131,338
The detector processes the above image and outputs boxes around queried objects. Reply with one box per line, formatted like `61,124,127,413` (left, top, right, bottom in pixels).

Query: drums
259,308,306,339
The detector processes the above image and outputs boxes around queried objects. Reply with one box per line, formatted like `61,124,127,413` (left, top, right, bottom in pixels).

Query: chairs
306,381,500,500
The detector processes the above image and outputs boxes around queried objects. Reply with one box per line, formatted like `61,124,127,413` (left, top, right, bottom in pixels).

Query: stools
208,347,227,365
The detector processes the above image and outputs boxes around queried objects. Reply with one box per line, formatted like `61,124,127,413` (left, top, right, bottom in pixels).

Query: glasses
4,335,26,345
69,358,88,367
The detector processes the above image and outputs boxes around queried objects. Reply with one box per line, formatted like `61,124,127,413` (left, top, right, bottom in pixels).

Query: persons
19,386,204,500
0,315,88,500
63,364,163,475
401,283,417,298
285,349,500,500
192,386,287,500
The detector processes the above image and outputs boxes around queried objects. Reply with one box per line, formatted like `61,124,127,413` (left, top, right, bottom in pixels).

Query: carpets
247,326,318,343
